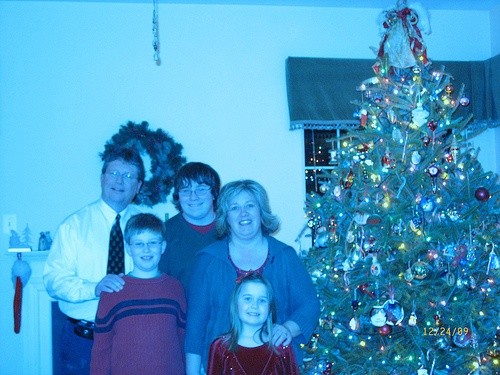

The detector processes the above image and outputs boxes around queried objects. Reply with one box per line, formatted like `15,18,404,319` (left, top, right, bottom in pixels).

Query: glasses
127,240,163,249
178,186,212,195
100,170,141,180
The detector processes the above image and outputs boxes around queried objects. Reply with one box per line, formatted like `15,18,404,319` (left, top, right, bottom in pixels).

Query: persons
157,162,230,312
183,179,320,375
89,213,190,375
206,269,300,375
42,147,161,375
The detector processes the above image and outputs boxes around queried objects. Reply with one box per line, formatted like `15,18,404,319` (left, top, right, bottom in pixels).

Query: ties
106,214,125,278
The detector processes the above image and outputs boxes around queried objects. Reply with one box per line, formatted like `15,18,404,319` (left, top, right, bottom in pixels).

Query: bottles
46,231,53,249
38,232,47,250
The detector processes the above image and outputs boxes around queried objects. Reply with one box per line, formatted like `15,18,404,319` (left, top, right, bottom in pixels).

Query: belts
65,316,97,327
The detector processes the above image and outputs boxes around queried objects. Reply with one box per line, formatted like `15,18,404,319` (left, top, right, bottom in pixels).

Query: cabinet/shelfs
19,251,53,375
